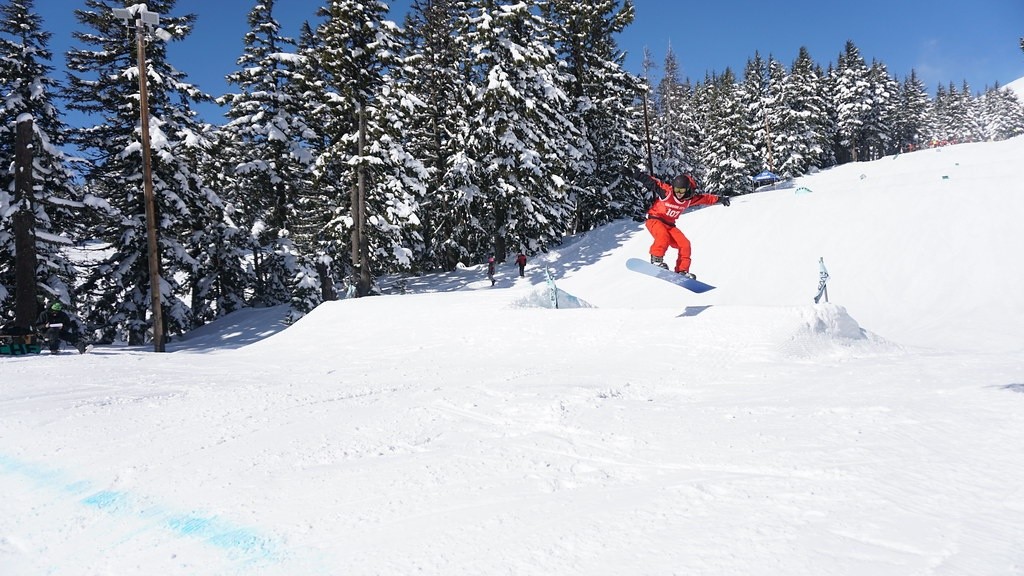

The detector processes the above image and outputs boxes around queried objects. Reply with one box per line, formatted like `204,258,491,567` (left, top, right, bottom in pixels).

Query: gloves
717,194,731,209
616,164,633,177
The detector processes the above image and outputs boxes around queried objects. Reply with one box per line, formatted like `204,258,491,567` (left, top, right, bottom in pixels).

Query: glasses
673,186,688,194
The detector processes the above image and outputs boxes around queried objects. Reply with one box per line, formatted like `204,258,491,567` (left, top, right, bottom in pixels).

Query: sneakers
80,344,94,355
652,259,668,270
676,272,697,281
50,347,59,354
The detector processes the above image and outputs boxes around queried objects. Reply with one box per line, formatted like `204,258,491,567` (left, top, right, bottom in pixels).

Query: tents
752,168,780,191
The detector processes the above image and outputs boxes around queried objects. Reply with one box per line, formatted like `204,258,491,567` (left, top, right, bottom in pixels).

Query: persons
621,164,732,280
486,257,497,286
514,250,526,276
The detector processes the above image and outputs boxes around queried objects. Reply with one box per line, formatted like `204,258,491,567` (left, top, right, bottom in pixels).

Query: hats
51,302,62,312
672,175,691,192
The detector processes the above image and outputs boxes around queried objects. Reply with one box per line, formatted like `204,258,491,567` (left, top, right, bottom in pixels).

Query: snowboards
625,258,716,293
